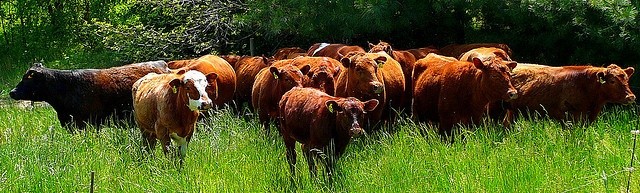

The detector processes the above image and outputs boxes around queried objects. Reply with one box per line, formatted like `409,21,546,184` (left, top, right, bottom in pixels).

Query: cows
271,47,307,60
278,86,380,178
82,64,165,131
440,43,512,60
167,55,241,70
339,51,406,135
233,54,288,115
131,69,218,169
306,43,367,62
9,62,119,136
174,54,237,109
290,55,344,97
457,46,514,130
367,41,417,122
406,47,443,60
252,64,311,136
132,59,171,74
502,63,636,132
409,54,519,144
